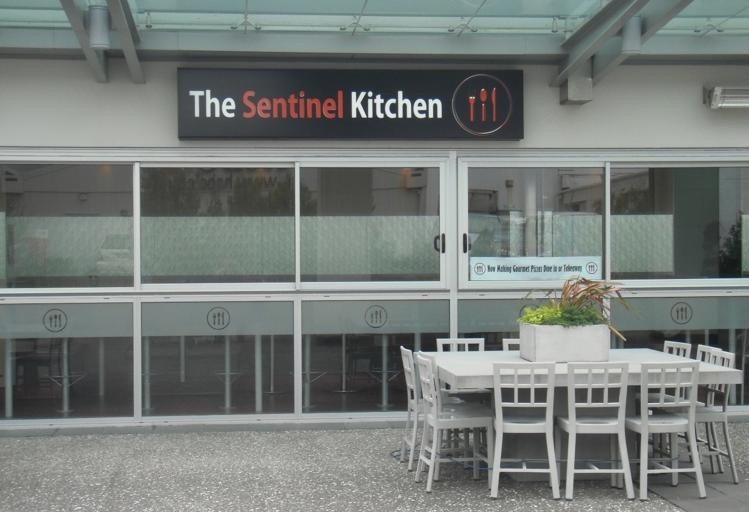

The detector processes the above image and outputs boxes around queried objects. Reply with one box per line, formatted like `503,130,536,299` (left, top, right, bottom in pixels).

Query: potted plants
515,275,634,363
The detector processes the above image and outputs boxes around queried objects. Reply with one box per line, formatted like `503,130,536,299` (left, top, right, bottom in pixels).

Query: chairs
401,338,743,499
